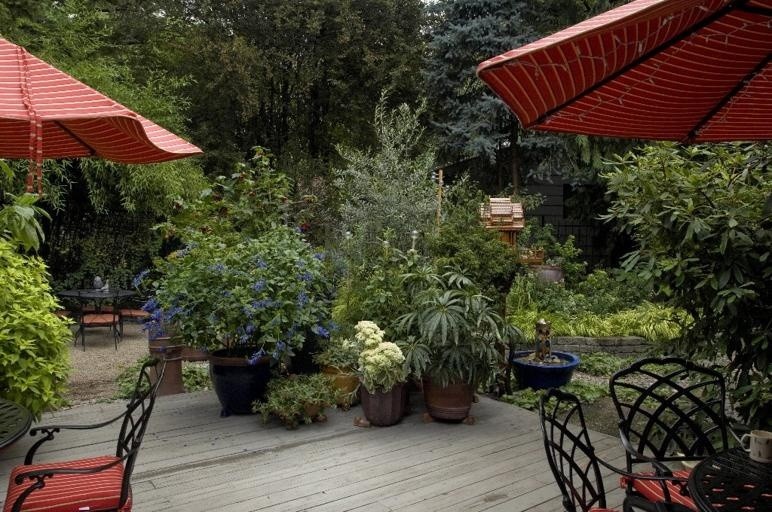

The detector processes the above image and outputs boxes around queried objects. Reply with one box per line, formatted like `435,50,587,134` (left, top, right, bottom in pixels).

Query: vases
506,350,580,392
360,379,412,426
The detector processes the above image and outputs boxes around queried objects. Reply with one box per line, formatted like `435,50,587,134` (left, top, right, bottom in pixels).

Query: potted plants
172,305,213,361
176,230,282,411
328,248,365,408
291,371,331,415
142,273,188,397
413,269,487,427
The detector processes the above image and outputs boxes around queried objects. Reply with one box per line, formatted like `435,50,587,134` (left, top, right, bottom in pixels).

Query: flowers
350,316,413,374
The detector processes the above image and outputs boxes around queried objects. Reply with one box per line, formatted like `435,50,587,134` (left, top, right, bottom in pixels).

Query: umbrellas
470,0,772,148
0,34,206,204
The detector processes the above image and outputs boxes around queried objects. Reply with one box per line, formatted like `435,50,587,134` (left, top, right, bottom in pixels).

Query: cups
740,429,772,464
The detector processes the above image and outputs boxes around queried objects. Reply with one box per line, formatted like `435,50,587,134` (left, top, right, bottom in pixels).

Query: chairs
4,355,168,512
609,356,729,512
539,388,689,512
56,289,151,349
